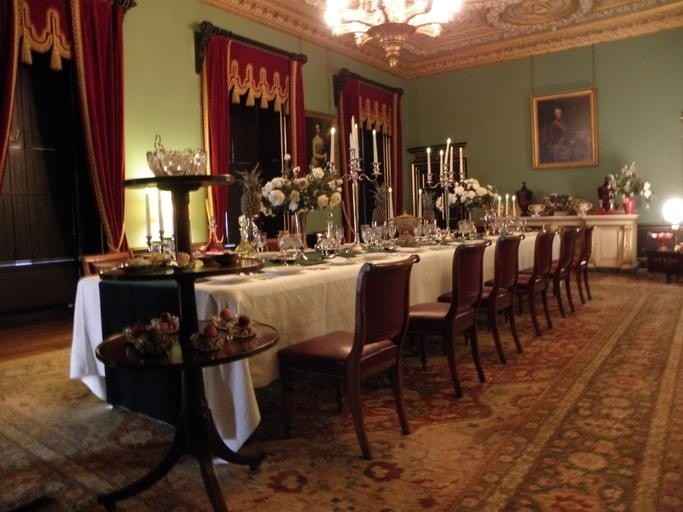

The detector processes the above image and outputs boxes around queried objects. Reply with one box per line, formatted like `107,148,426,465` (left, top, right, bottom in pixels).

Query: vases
622,195,634,214
553,210,567,216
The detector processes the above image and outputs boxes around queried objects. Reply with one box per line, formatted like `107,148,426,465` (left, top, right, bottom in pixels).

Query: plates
258,265,304,277
119,250,241,273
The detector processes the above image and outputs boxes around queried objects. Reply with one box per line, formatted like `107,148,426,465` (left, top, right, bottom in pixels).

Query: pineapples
422,190,436,224
237,164,261,218
370,181,389,226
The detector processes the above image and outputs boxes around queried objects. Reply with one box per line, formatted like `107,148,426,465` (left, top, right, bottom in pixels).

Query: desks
643,246,683,282
515,214,639,274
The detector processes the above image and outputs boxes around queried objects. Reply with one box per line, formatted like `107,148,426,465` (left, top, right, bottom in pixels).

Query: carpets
0,280,681,510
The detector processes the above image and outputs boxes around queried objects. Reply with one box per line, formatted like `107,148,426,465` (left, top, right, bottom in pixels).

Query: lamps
318,0,464,71
663,197,683,248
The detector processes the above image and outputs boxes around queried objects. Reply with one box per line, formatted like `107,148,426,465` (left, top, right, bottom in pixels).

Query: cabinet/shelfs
94,174,279,511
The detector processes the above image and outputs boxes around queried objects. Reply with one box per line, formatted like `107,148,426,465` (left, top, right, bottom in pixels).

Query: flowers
606,162,660,209
437,180,501,218
544,191,574,209
257,163,343,216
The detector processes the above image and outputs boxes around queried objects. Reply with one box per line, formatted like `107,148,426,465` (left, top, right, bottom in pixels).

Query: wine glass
240,217,530,259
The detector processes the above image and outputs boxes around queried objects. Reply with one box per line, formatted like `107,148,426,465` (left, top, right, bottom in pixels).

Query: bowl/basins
145,149,209,177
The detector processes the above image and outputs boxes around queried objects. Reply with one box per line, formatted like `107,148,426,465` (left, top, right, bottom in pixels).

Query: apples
239,316,250,327
159,322,172,330
205,326,218,337
132,322,146,334
220,309,233,321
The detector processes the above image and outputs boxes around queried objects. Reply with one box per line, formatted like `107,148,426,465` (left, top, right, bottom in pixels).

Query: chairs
78,250,131,274
384,223,593,402
276,250,418,462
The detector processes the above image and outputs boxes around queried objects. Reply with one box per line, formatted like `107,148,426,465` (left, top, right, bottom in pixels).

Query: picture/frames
530,87,597,170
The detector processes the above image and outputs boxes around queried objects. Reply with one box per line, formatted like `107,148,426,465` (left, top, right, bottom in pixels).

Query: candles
496,195,515,218
388,188,394,219
423,138,464,185
326,116,380,168
418,191,422,218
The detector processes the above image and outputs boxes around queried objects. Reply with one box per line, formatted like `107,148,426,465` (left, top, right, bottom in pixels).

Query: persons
546,105,586,161
307,122,327,173
